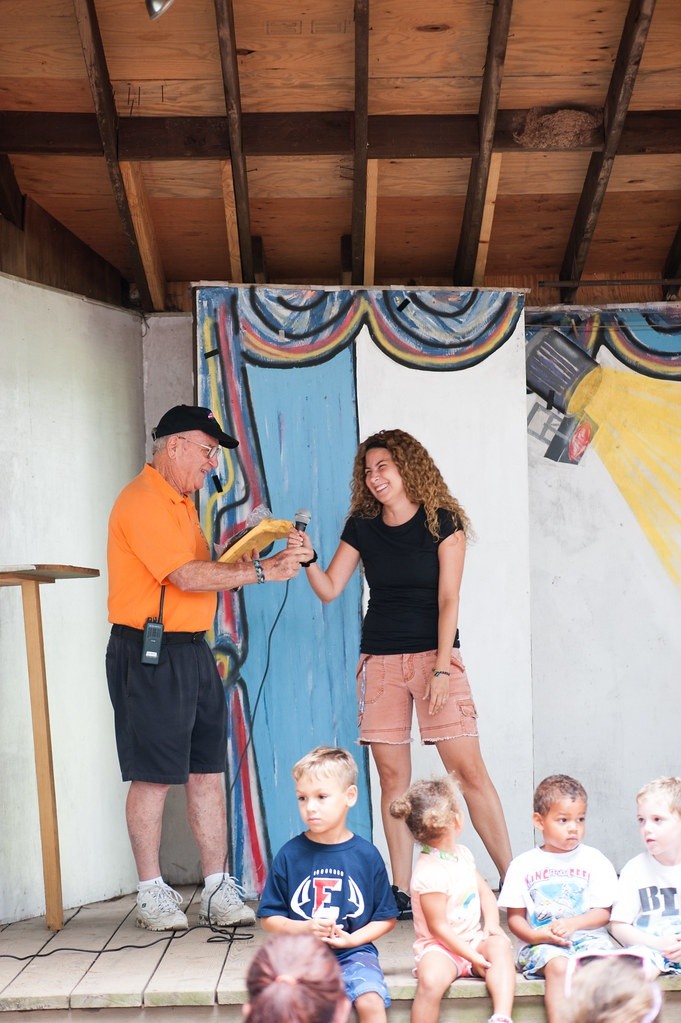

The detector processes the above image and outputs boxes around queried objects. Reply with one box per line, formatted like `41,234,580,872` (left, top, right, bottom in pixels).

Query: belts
111,626,204,645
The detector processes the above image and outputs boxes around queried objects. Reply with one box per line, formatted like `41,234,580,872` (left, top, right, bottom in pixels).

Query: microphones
295,508,311,532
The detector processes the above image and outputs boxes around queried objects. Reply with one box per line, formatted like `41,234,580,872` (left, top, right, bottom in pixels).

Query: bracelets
300,549,318,567
253,559,265,584
432,669,450,676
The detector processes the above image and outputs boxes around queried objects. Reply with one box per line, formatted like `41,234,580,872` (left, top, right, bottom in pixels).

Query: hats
151,404,239,449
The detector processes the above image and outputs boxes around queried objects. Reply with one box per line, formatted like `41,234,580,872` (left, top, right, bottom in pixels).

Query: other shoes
499,881,503,893
488,1015,512,1023
389,884,415,921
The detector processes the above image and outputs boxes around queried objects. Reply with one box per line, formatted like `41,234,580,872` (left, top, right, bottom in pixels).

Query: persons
107,404,312,933
389,780,518,1023
608,777,681,970
496,774,618,1023
241,933,352,1023
288,431,514,915
256,745,398,1023
569,946,663,1023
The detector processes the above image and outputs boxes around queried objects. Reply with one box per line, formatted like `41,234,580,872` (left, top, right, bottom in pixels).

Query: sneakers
135,883,189,931
199,876,256,926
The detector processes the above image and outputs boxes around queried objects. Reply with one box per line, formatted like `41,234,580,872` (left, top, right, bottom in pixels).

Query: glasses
177,436,221,459
563,949,665,988
